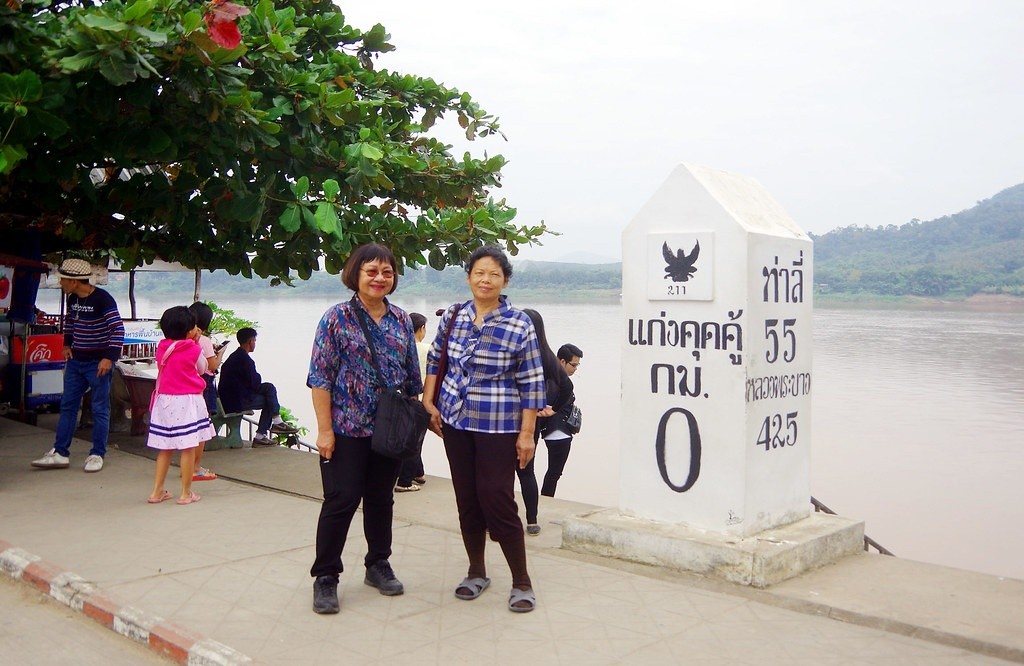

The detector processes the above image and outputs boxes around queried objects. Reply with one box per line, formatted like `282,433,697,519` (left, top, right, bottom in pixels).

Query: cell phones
215,340,231,352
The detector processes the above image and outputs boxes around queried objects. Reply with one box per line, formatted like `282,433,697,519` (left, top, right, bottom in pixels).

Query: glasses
361,268,396,278
567,360,580,367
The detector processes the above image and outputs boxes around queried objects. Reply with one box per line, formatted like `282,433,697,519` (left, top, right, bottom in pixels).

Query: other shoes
395,484,420,491
527,524,540,534
414,476,425,483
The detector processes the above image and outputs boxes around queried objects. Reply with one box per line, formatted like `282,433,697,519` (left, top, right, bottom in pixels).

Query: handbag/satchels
563,405,582,434
370,390,432,460
148,389,158,412
427,304,462,433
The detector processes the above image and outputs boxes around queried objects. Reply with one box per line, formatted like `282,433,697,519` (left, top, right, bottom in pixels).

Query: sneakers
252,436,278,447
30,448,70,467
313,576,339,613
84,455,104,472
365,558,403,595
270,422,299,434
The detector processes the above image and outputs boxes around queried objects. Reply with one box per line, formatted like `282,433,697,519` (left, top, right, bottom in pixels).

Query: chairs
203,397,255,450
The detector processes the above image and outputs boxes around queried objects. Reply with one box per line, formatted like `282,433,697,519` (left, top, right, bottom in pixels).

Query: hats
54,258,94,279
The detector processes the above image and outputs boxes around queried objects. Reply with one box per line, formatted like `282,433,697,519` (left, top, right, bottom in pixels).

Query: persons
422,246,547,611
515,309,574,535
395,313,429,493
217,327,299,448
306,243,425,613
146,306,217,504
31,259,125,472
188,301,217,480
541,344,583,497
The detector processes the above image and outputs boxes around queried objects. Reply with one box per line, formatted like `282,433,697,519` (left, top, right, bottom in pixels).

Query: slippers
455,577,490,599
177,491,201,504
192,467,217,480
509,587,534,612
148,490,172,503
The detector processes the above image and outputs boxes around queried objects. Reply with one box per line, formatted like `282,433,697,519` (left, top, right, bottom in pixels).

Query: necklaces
75,284,92,320
